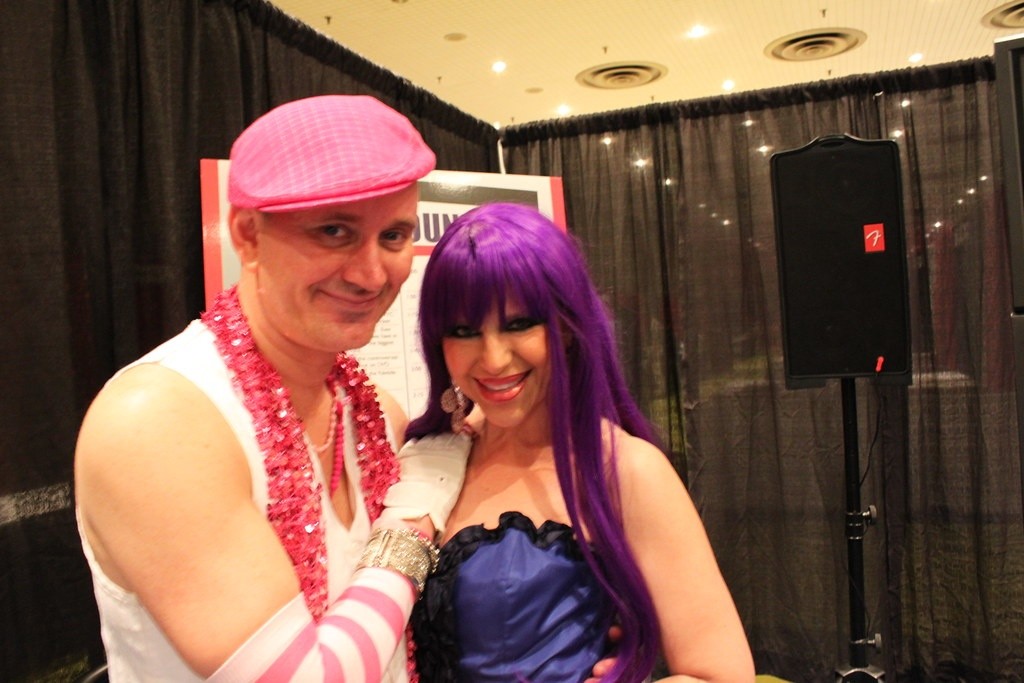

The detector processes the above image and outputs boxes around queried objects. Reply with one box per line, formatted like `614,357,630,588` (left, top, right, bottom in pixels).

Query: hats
228,94,437,213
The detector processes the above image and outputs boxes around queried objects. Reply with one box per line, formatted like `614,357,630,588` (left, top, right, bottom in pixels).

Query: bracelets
354,528,439,594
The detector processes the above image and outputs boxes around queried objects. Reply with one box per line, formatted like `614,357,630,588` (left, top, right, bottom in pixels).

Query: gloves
382,433,471,536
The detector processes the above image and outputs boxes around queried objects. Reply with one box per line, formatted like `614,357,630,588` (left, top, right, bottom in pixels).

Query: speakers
768,132,912,381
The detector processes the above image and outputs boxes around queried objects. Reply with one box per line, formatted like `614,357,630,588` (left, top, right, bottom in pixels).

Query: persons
75,95,621,683
402,202,754,683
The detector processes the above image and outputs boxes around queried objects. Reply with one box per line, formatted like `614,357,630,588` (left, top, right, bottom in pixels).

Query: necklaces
315,376,345,498
